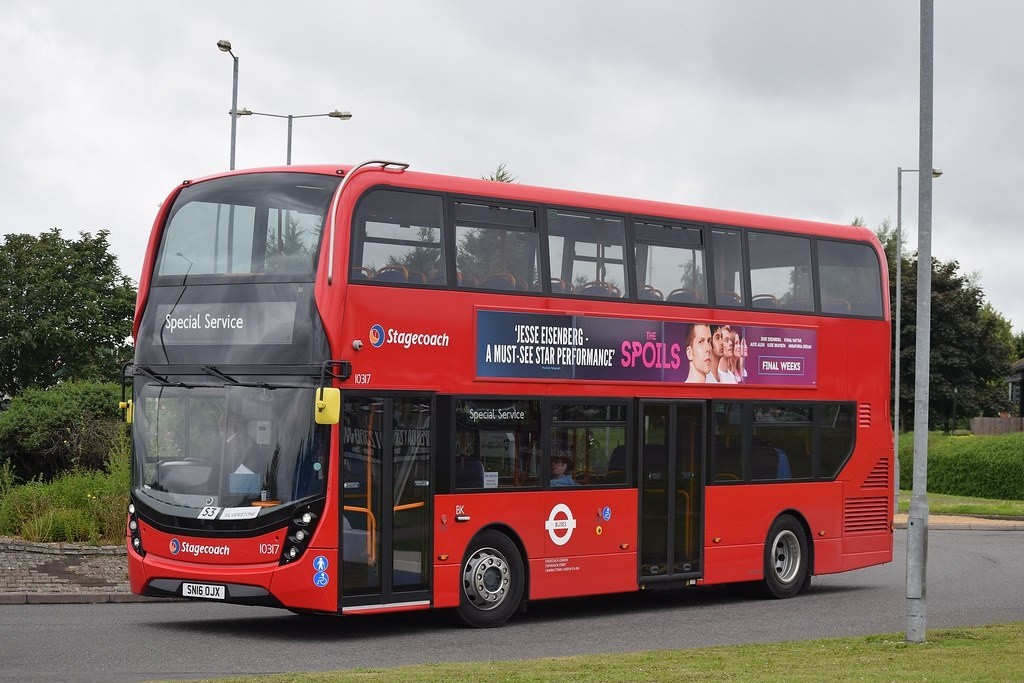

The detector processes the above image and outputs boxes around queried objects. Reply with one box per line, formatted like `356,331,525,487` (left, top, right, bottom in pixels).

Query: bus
120,160,894,628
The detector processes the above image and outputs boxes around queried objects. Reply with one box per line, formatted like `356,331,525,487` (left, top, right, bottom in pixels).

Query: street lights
216,39,239,274
893,167,943,517
229,109,352,254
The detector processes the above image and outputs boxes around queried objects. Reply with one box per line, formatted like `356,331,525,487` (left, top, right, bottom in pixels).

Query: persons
550,452,575,487
685,323,748,383
211,411,258,474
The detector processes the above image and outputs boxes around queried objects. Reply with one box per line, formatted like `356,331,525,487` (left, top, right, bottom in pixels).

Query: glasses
551,458,565,464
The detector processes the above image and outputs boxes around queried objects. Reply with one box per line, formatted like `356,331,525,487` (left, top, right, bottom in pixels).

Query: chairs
574,435,810,486
352,265,852,313
